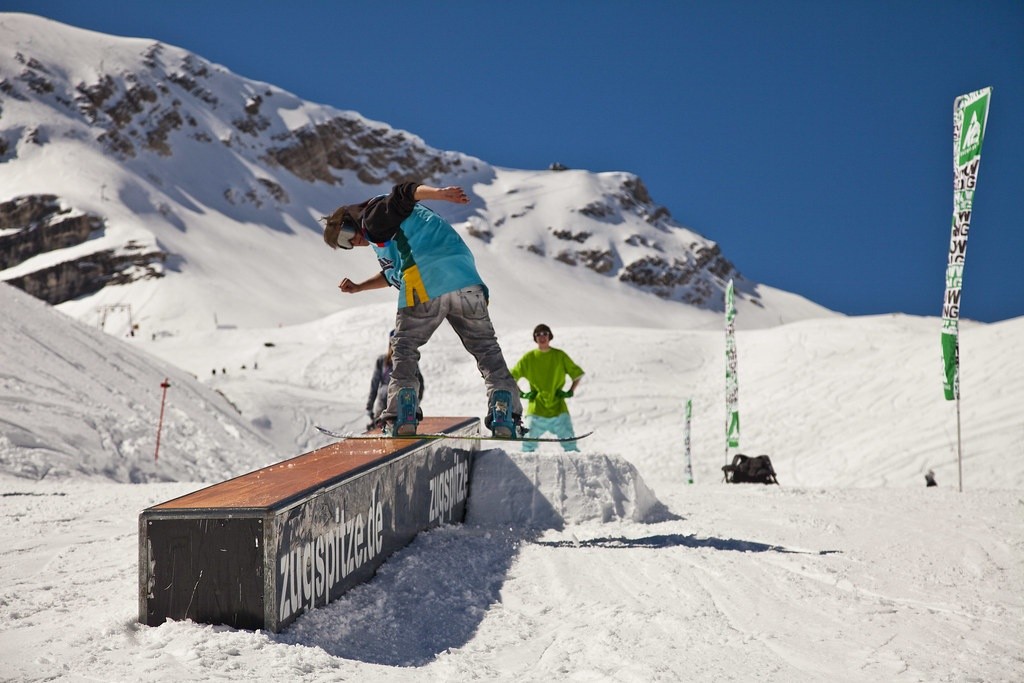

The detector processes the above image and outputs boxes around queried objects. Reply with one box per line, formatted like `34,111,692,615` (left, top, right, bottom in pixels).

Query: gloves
556,390,574,399
520,390,537,400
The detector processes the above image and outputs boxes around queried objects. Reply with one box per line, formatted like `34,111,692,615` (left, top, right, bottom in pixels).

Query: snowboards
316,424,594,442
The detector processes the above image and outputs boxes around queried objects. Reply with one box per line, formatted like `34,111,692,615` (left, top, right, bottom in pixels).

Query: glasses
535,332,549,337
336,207,357,249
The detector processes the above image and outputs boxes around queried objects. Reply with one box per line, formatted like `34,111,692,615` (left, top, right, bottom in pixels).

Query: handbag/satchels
721,453,778,485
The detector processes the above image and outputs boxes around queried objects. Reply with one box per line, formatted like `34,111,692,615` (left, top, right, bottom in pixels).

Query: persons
366,328,424,426
509,324,586,452
318,181,523,439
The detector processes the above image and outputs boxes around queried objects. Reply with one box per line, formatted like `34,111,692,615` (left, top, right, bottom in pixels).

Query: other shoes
491,416,522,438
386,417,417,435
